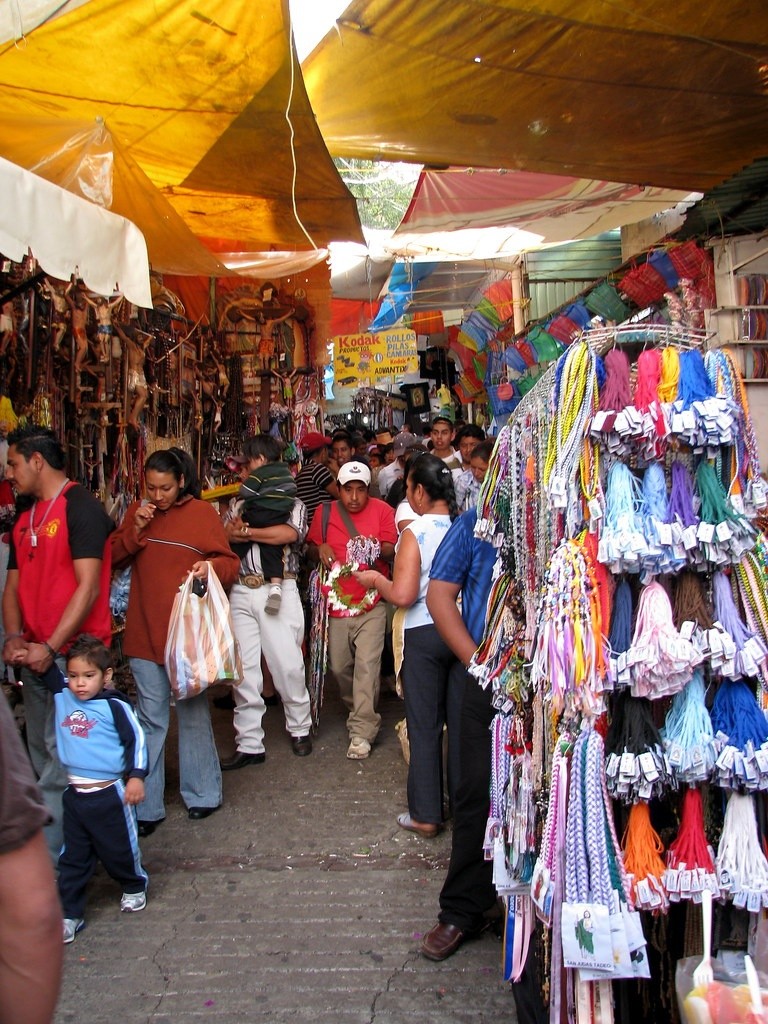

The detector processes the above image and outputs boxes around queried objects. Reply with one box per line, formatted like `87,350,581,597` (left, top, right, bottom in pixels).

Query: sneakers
120,891,147,913
63,918,85,943
346,736,371,759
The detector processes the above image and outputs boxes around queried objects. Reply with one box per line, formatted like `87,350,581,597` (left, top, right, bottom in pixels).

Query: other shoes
264,594,281,614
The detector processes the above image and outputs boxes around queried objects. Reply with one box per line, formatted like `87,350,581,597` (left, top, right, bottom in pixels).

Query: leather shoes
136,816,167,837
221,751,266,771
420,921,463,961
291,736,313,756
188,804,221,820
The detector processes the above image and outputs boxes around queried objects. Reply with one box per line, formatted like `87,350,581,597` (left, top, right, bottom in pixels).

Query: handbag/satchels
163,561,239,701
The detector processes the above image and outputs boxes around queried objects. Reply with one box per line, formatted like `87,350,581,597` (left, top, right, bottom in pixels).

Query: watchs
241,526,249,533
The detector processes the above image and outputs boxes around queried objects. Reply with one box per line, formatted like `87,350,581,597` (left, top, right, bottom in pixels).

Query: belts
235,572,297,590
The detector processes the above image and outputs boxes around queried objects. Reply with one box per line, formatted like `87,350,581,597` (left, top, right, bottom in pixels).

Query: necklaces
29,478,69,547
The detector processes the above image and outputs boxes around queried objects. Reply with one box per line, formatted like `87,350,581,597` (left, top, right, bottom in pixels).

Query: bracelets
373,573,383,588
43,642,57,658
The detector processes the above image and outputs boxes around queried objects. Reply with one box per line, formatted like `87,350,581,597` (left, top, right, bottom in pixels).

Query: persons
0,686,63,1024
0,276,296,431
0,418,501,959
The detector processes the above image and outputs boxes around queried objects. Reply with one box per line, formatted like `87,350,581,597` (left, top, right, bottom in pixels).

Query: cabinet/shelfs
710,305,768,383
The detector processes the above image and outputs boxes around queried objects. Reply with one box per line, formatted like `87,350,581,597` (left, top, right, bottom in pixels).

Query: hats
392,432,416,457
376,431,395,444
226,456,249,473
300,431,331,452
336,461,372,485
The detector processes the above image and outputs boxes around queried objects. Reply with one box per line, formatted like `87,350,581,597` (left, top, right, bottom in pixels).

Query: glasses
369,452,380,457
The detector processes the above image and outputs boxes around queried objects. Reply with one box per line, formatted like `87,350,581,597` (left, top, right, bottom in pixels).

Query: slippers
397,812,438,838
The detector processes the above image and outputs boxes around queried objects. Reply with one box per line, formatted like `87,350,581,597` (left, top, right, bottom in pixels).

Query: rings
328,558,332,562
